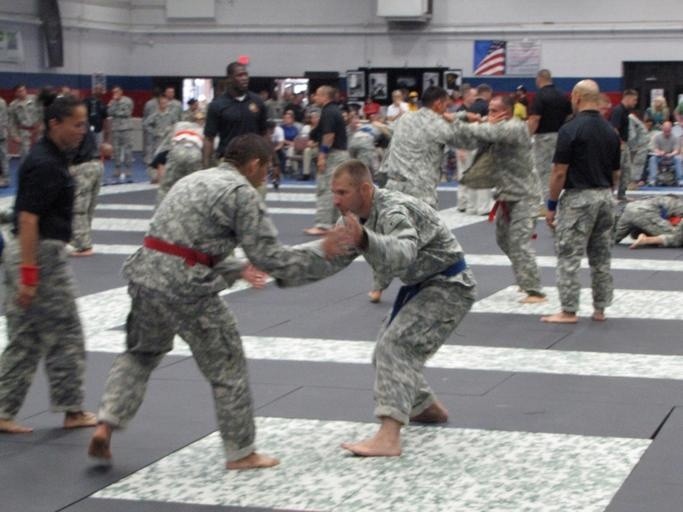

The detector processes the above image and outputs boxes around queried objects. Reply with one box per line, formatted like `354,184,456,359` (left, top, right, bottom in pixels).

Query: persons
176,99,201,119
274,108,303,177
106,88,136,183
272,123,283,158
516,86,529,103
144,99,178,182
387,88,407,119
270,161,476,456
445,87,467,117
264,120,282,188
0,98,12,189
2,95,97,432
299,110,322,176
444,95,546,304
90,132,355,470
84,85,107,164
630,193,682,250
203,62,270,209
539,80,622,324
10,85,39,163
146,87,163,165
644,97,668,131
650,119,683,188
512,97,526,121
454,86,476,216
672,94,683,133
338,101,389,139
305,88,350,236
69,125,104,255
526,66,569,217
627,113,648,191
148,120,208,218
366,84,508,300
146,87,179,116
606,88,640,204
613,196,679,248
469,87,498,216
